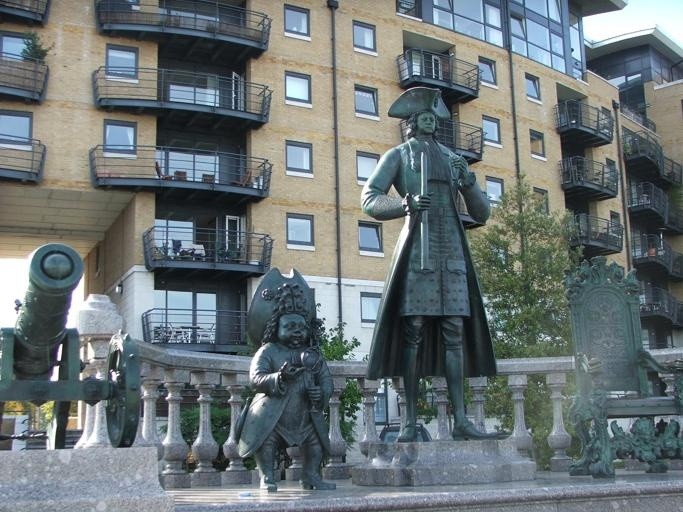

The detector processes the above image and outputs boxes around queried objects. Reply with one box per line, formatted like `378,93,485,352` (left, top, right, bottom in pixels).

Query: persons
231,311,339,491
359,109,500,443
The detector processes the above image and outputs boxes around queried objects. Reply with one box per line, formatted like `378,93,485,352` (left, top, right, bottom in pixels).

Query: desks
179,325,199,344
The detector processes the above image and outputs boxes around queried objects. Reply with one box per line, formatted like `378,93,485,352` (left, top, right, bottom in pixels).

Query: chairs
198,323,214,343
562,257,682,479
168,321,184,343
154,161,172,181
229,169,251,188
